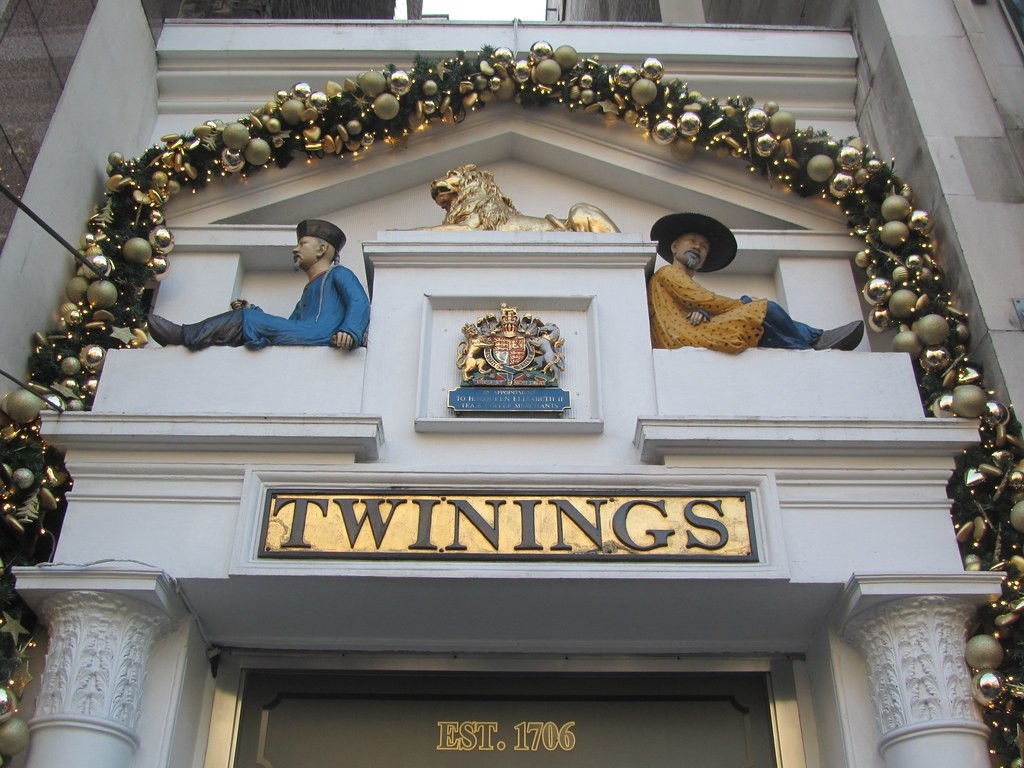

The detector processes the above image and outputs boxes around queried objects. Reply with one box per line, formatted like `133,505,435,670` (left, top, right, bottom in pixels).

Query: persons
649,213,864,354
146,219,371,350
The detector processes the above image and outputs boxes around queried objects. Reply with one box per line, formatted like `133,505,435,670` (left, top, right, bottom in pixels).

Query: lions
383,163,621,233
